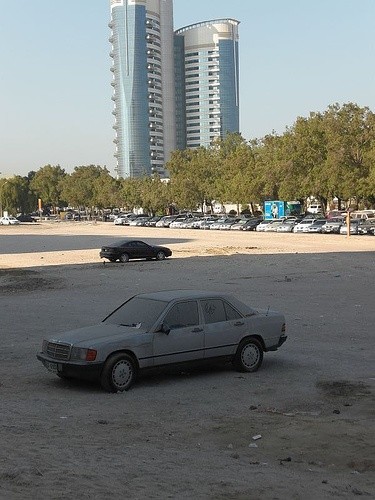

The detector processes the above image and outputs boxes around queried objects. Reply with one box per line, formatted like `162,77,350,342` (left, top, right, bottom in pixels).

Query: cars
99,239,172,263
221,218,240,229
36,287,288,394
307,219,328,233
257,219,273,231
242,219,263,230
110,208,216,229
357,220,375,234
340,218,365,235
323,216,351,236
209,217,228,230
293,218,316,232
266,223,279,232
16,214,37,222
278,221,294,233
325,210,345,220
0,216,19,225
231,219,247,231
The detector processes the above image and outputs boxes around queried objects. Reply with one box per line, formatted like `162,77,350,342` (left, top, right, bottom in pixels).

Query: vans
349,211,375,221
306,204,323,214
